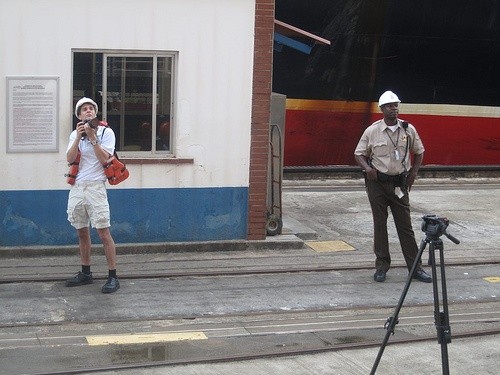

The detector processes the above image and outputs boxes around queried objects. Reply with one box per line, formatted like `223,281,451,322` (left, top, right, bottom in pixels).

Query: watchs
90,138,98,146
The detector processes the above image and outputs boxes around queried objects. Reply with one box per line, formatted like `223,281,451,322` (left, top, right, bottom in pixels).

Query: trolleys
266,123,285,236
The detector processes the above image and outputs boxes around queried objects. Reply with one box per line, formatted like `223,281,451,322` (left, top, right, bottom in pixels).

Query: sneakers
374,271,386,282
102,278,120,293
67,272,93,287
413,270,433,282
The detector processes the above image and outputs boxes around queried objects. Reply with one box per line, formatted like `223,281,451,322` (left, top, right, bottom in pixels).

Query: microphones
402,121,408,131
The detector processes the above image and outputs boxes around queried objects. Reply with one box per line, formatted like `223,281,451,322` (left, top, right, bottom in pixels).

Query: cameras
78,117,100,137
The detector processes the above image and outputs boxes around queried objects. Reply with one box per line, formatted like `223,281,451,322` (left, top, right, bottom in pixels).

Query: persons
66,97,120,294
354,91,432,283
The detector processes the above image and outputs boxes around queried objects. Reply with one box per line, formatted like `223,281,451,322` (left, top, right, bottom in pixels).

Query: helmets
75,96,98,118
378,91,401,107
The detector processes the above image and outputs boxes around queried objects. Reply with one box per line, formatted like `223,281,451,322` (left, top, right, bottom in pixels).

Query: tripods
367,235,452,374
